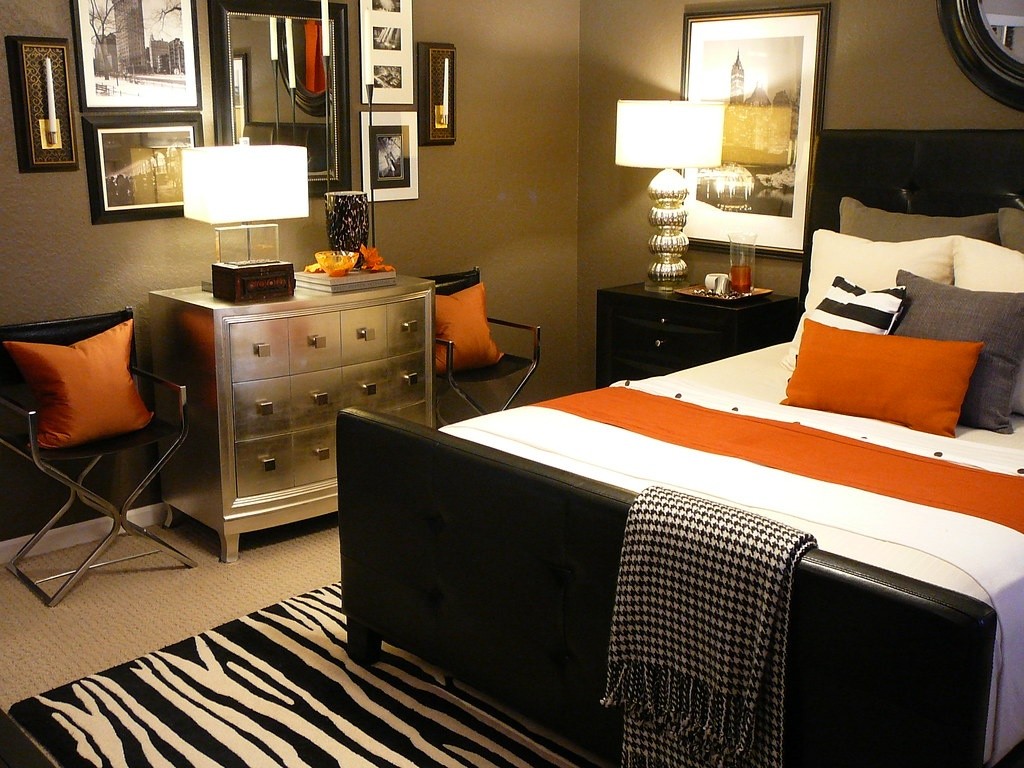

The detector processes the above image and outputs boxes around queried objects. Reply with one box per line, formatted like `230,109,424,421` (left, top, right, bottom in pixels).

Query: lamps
614,99,724,294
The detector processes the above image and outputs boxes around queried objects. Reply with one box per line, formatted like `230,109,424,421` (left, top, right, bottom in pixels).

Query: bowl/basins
315,250,359,276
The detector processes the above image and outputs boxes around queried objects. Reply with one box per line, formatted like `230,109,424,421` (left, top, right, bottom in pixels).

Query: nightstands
596,279,804,389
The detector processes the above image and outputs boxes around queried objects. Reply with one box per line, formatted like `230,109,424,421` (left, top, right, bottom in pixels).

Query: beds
336,127,1024,768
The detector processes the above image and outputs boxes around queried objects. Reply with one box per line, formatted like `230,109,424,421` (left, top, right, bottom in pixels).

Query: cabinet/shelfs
148,266,432,562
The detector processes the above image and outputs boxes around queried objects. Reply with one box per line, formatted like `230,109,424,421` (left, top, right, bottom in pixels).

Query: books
295,268,397,292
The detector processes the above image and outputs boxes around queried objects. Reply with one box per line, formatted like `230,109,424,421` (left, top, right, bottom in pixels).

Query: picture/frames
71,0,204,112
360,0,414,104
81,114,205,225
680,3,831,261
360,111,419,202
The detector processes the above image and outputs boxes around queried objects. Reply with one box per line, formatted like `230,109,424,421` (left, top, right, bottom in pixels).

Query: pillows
2,320,154,449
434,282,505,376
781,196,1024,439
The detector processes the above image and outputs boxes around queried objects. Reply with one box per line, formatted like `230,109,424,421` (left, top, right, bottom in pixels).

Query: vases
208,0,351,197
325,190,370,270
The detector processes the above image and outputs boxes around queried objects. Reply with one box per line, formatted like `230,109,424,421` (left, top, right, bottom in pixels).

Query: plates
674,285,773,302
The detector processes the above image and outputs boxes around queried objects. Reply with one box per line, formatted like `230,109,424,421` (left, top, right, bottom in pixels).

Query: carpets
11,579,619,768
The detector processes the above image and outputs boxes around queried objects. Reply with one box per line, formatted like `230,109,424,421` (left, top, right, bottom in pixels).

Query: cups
704,274,729,291
729,233,756,294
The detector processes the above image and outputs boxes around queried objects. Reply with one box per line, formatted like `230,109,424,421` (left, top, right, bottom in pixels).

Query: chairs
1,308,199,608
421,266,545,427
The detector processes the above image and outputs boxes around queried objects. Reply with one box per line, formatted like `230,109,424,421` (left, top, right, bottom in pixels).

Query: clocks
936,0,1024,112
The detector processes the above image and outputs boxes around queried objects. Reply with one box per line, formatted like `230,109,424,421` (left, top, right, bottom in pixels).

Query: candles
443,57,448,124
45,55,57,132
